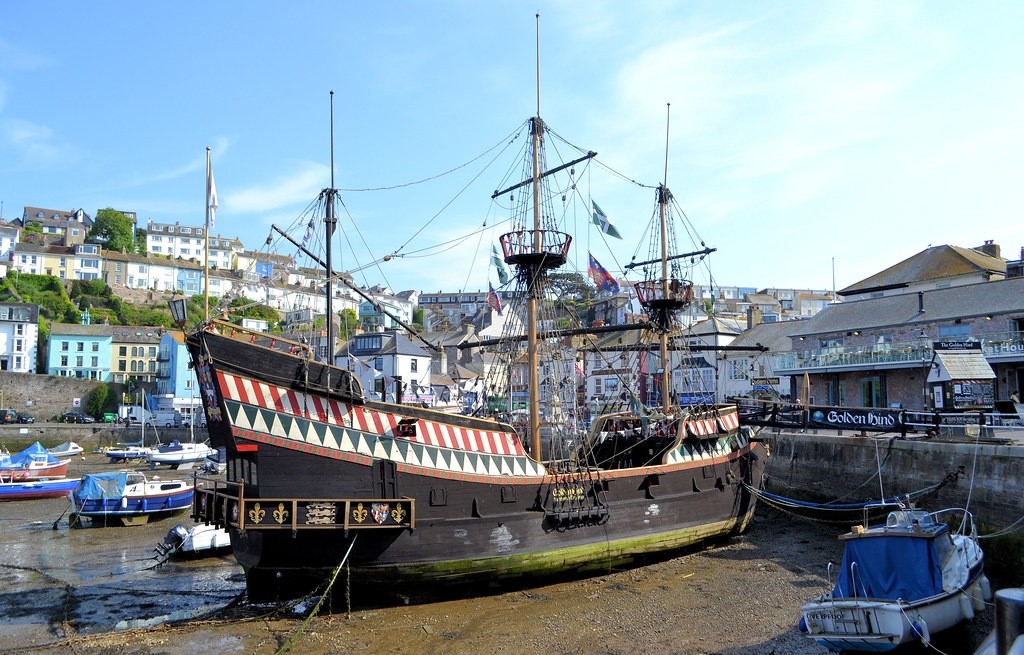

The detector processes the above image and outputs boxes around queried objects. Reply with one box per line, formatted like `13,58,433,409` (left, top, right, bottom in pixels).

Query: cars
61,411,95,424
15,412,35,425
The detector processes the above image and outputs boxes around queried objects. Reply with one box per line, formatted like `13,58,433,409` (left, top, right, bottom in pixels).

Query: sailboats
178,9,771,608
99,385,227,476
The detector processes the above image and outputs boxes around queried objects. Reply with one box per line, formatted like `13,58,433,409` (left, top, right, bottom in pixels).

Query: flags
492,244,508,285
588,253,619,293
486,282,503,316
591,199,622,240
206,158,218,229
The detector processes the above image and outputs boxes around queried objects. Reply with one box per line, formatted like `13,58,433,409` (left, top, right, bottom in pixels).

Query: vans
184,413,207,429
145,411,182,428
117,404,151,423
0,409,18,424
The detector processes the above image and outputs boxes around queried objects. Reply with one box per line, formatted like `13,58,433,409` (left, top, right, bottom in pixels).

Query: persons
1010,391,1020,404
795,398,801,404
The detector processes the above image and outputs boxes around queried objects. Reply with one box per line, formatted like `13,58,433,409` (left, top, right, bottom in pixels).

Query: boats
45,440,85,459
0,476,82,500
153,520,233,556
796,491,993,655
67,469,206,527
0,441,72,480
